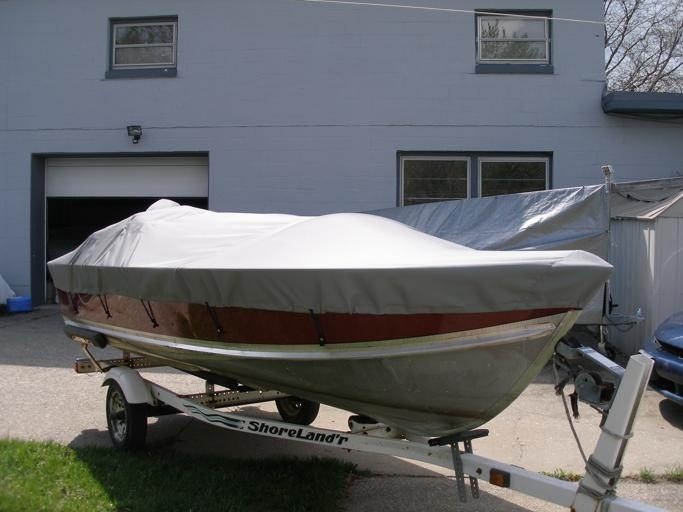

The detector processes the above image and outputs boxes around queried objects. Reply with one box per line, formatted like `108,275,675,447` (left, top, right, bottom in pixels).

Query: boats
45,199,615,440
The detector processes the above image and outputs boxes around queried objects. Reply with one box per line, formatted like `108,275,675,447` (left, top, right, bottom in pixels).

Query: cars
644,310,682,414
